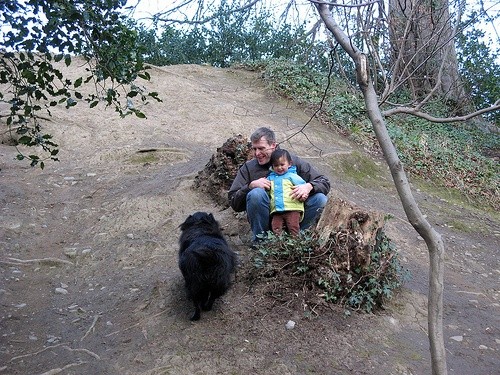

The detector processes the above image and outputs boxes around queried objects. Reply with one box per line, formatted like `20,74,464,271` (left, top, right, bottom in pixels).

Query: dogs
178,211,239,322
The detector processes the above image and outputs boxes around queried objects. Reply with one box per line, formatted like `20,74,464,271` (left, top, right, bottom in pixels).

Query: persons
264,148,309,239
228,127,332,242
